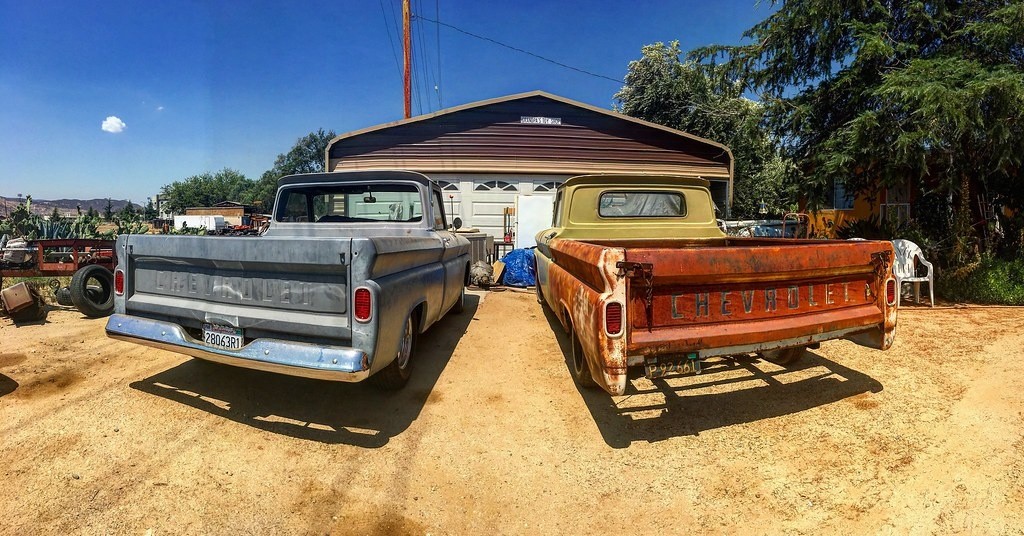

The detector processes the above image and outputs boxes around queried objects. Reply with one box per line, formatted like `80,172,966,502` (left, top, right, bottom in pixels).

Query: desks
495,241,514,262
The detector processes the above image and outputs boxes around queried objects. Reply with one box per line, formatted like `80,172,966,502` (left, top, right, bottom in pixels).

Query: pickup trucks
104,169,475,391
532,169,905,397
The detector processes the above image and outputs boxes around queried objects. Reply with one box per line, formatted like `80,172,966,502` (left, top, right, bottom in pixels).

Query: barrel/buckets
3,238,27,264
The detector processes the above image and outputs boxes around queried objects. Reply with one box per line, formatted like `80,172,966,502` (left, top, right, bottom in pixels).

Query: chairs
888,239,934,308
